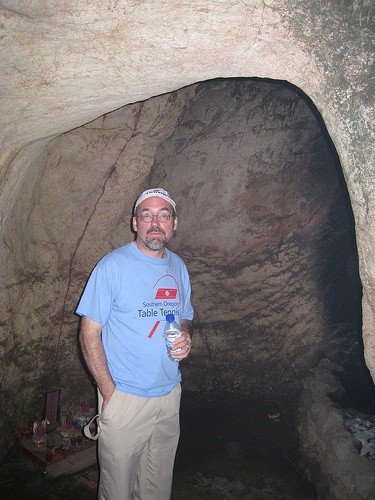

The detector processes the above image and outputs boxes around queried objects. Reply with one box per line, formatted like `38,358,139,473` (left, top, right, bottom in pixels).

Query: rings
185,346,189,352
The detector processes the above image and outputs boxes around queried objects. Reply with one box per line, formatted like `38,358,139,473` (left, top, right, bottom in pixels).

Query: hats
134,188,177,214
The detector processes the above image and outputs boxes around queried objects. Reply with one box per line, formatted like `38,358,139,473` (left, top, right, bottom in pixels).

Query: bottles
165,314,184,362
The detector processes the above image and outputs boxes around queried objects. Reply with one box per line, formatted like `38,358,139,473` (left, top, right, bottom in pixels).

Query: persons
73,183,198,500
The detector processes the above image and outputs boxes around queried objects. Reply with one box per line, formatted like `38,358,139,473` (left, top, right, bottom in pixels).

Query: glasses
137,214,173,223
